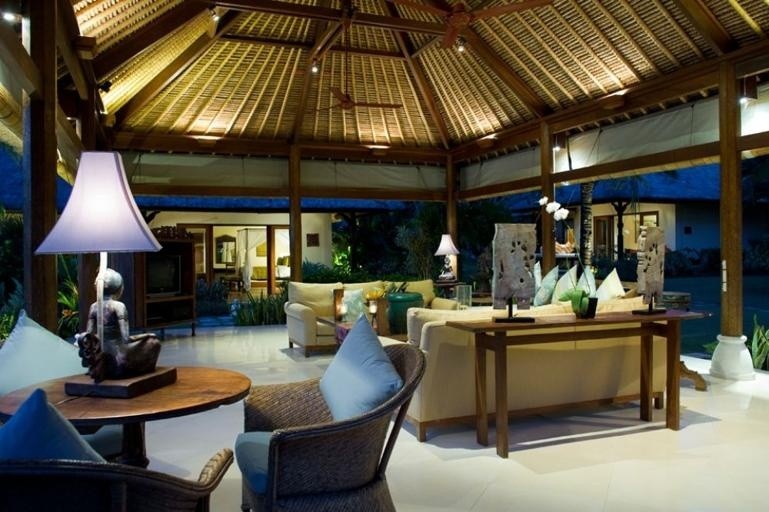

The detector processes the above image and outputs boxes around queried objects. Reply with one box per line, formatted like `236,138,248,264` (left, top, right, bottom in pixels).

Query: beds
238,266,290,279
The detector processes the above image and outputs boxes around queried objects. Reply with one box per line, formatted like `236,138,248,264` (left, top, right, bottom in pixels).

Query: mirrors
215,234,236,264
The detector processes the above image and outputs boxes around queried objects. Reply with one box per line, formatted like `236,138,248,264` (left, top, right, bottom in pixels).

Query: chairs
241,343,428,512
1,446,233,511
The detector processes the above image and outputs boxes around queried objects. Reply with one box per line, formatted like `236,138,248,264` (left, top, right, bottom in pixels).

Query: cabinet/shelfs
185,227,206,274
135,225,197,337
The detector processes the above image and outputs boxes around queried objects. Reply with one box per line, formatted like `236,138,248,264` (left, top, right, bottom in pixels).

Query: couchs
284,279,461,358
378,295,664,443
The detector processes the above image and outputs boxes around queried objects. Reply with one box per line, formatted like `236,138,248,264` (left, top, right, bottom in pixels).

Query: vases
572,294,596,319
365,300,379,327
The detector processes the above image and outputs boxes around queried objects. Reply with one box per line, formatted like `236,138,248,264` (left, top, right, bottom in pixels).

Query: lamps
36,150,177,397
433,234,459,283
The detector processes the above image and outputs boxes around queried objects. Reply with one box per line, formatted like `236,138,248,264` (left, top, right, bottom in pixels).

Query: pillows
577,266,596,298
536,264,556,309
551,264,577,305
4,387,115,463
596,267,626,300
532,259,542,292
0,308,89,394
317,313,402,424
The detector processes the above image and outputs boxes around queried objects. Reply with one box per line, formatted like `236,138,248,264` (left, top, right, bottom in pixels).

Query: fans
306,24,406,115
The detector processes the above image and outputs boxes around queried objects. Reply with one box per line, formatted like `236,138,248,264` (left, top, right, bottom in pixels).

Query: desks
1,368,251,479
447,308,661,454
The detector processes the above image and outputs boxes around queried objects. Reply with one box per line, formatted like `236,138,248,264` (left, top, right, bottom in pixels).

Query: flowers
536,196,598,319
363,288,384,307
